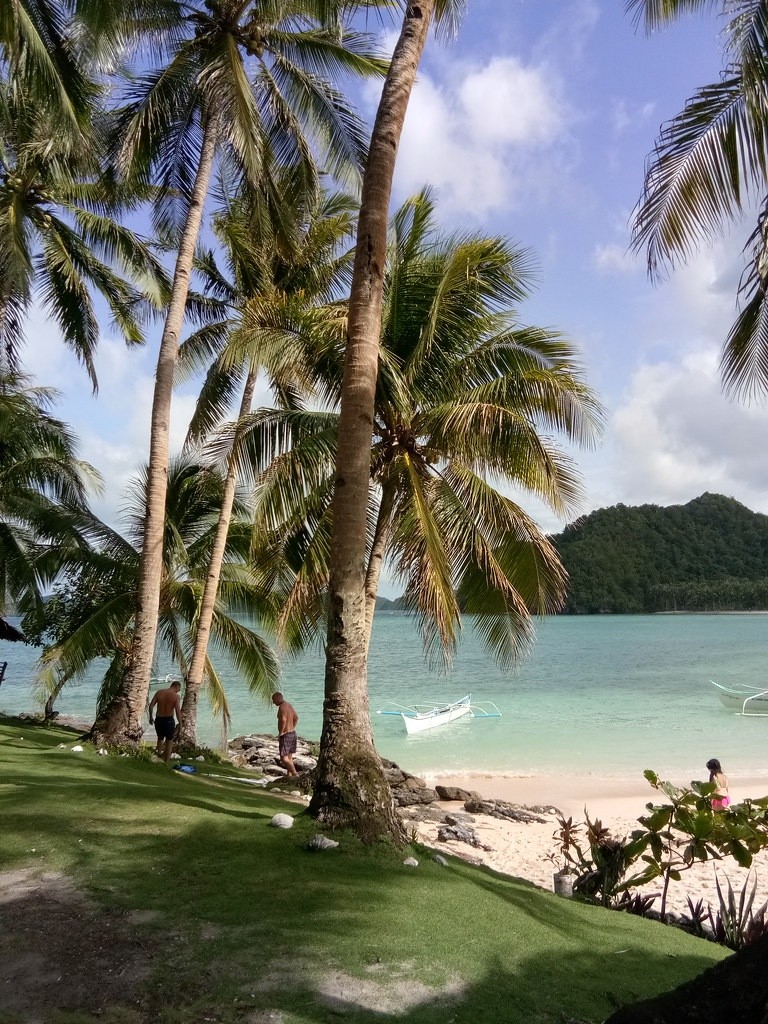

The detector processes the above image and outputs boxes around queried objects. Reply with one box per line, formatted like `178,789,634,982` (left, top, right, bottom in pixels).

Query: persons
272,692,300,778
706,758,731,811
149,681,182,762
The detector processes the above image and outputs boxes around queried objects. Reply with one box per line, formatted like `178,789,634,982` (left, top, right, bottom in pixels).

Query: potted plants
541,813,611,899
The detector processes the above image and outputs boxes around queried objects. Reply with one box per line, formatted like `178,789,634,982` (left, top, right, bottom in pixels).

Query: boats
708,678,768,717
376,692,503,734
